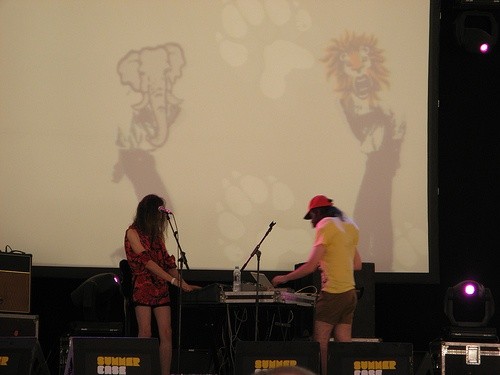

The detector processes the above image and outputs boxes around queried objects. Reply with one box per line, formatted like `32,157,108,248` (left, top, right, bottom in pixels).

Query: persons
126,193,202,375
71,258,130,320
271,194,363,375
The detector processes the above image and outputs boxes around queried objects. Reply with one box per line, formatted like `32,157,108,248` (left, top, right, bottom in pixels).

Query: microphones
157,206,173,214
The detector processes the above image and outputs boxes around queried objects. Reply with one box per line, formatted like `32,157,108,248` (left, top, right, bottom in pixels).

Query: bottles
233,266,241,292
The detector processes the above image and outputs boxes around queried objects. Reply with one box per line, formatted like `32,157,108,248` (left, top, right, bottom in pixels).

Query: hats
304,195,333,220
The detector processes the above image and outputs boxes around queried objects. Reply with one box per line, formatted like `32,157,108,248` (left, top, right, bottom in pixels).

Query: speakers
68,322,123,338
70,337,162,375
233,340,321,375
0,270,32,315
327,342,415,375
0,338,50,375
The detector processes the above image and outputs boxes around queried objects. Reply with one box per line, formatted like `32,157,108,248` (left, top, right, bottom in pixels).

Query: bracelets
171,277,175,285
